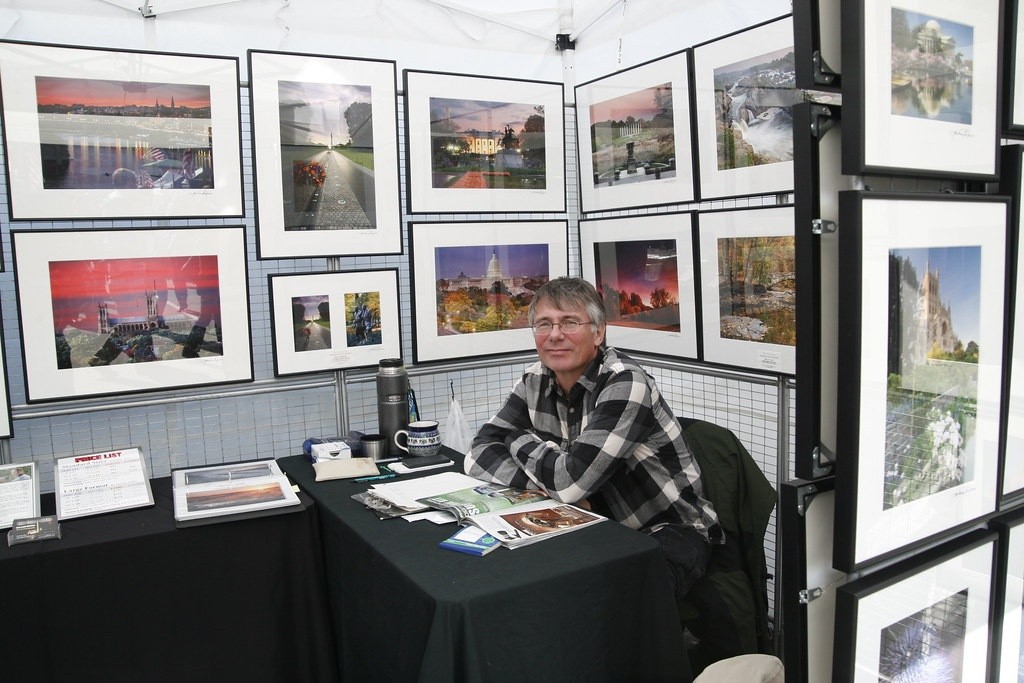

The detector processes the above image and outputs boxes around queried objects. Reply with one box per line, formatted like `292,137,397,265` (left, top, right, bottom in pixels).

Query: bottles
376,359,409,455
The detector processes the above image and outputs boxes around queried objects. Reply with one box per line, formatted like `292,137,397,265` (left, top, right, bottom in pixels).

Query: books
439,523,503,557
414,482,608,551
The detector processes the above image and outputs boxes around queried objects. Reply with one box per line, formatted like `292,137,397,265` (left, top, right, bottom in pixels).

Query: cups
394,420,441,457
360,434,387,460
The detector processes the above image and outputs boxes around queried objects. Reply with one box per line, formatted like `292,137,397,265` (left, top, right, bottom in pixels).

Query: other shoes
177,304,188,312
88,356,110,366
182,350,200,358
159,324,169,330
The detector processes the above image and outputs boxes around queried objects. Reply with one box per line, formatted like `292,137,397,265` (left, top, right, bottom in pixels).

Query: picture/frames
694,203,797,385
989,507,1024,683
830,527,1001,683
576,210,700,367
265,265,406,381
242,47,406,263
0,38,246,221
687,10,799,202
402,65,571,217
10,220,258,406
833,188,1007,574
573,41,695,216
841,0,1012,183
408,219,572,365
779,0,843,683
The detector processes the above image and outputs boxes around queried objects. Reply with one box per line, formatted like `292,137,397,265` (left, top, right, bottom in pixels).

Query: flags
136,146,193,189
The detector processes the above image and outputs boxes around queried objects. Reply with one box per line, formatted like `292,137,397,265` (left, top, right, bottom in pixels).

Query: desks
0,458,316,683
276,443,693,683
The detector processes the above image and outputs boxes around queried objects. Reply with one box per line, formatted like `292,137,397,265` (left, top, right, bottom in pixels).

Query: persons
354,304,374,345
464,277,726,602
151,278,222,358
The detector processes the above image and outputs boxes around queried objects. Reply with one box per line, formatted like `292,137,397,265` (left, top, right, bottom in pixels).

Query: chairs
685,407,778,658
677,417,775,653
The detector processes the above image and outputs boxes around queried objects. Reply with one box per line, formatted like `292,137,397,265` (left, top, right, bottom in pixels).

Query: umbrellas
142,158,183,189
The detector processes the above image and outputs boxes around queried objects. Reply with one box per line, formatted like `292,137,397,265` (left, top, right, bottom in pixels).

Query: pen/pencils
350,473,400,483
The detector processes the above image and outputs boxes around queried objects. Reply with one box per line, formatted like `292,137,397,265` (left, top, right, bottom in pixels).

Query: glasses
532,319,596,335
